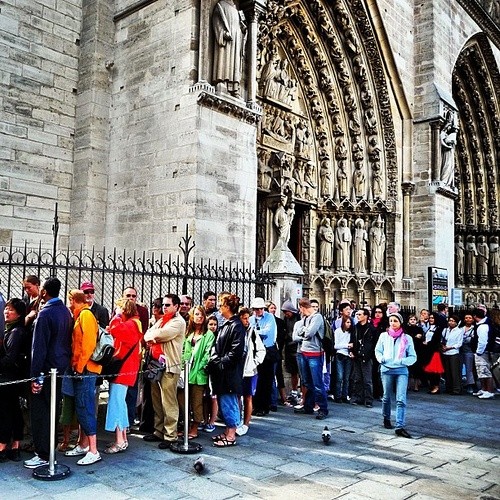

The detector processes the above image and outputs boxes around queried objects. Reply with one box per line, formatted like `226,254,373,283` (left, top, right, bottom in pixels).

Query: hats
251,298,267,309
80,282,94,291
388,312,403,324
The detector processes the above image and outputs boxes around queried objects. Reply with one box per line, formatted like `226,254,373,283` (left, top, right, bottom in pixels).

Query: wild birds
194,455,205,474
322,426,331,444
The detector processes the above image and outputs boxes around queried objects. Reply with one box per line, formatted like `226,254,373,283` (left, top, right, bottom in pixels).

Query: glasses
254,308,263,311
160,303,175,307
83,290,94,294
123,294,137,298
180,302,190,306
69,290,74,297
375,311,383,313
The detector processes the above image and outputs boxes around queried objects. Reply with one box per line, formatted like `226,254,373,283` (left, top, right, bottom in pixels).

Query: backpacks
304,313,335,357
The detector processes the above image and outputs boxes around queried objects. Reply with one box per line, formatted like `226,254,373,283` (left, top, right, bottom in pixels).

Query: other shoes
383,419,393,429
395,428,411,438
283,376,500,421
57,404,277,465
10,447,21,462
0,448,8,462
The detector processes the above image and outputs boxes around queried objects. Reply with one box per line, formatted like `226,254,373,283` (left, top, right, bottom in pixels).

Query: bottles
35,371,45,393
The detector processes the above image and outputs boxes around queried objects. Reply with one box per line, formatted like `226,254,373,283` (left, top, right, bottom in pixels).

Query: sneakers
23,452,57,468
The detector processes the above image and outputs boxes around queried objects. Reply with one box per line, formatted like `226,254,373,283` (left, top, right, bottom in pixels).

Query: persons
0,275,500,468
374,312,417,439
274,195,290,245
212,0,248,95
256,0,385,199
303,210,309,272
440,50,500,228
318,218,385,273
455,235,500,276
287,203,295,225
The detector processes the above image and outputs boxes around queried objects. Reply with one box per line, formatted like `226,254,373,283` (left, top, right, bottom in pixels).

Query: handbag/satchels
103,356,123,382
78,308,115,363
144,360,165,381
176,369,185,388
425,352,445,373
